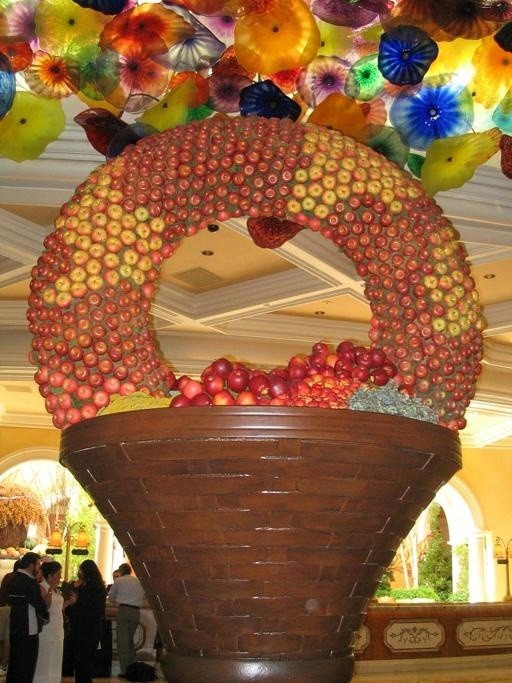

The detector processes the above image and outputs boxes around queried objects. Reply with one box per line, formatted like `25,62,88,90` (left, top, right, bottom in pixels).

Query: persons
1,550,147,682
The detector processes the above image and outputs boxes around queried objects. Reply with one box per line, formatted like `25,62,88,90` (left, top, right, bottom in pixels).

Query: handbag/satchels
125,662,156,682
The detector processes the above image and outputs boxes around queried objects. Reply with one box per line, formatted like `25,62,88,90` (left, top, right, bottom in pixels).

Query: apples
27,118,488,433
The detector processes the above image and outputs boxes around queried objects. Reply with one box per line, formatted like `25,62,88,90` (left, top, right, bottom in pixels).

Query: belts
120,604,139,608
